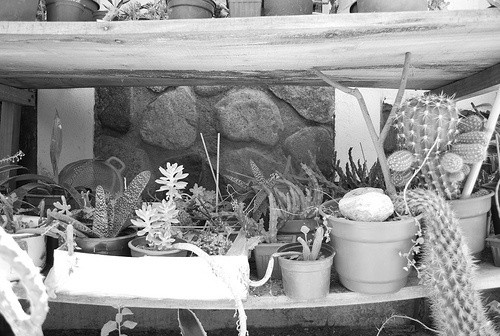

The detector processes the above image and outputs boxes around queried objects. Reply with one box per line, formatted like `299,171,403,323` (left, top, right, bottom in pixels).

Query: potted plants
263,0,314,16
319,186,421,296
394,88,499,264
254,192,290,282
44,0,85,21
354,0,430,14
0,0,40,22
80,0,101,21
47,169,151,257
276,225,335,301
0,190,51,273
227,0,263,17
166,0,217,19
265,163,325,243
127,161,191,258
